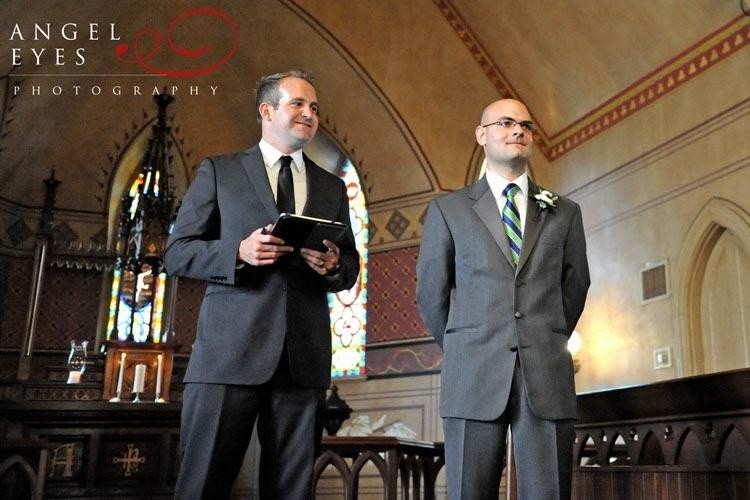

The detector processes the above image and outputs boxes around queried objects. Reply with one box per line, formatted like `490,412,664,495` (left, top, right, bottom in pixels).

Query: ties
277,156,296,214
502,183,523,270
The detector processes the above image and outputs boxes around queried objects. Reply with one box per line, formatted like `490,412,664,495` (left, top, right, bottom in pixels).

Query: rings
319,260,325,267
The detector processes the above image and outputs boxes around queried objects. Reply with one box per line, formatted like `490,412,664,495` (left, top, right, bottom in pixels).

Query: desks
309,437,445,500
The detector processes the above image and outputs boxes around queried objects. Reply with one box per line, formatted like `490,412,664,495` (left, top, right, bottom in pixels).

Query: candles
132,364,146,393
155,354,163,393
116,353,126,393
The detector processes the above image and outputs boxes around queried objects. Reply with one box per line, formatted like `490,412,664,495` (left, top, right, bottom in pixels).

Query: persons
415,99,593,500
163,70,360,500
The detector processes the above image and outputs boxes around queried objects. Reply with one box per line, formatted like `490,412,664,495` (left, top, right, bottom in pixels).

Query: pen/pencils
261,227,268,235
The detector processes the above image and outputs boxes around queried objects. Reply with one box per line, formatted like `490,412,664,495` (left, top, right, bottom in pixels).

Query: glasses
482,118,536,132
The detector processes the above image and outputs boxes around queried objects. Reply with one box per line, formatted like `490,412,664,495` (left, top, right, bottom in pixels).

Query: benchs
580,443,629,465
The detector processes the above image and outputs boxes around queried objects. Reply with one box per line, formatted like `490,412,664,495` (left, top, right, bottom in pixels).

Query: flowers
529,187,561,221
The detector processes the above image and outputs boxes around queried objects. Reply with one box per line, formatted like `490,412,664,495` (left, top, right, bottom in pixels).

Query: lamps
110,86,177,276
567,330,580,374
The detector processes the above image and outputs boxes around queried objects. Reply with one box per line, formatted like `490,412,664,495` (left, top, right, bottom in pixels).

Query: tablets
269,211,350,270
